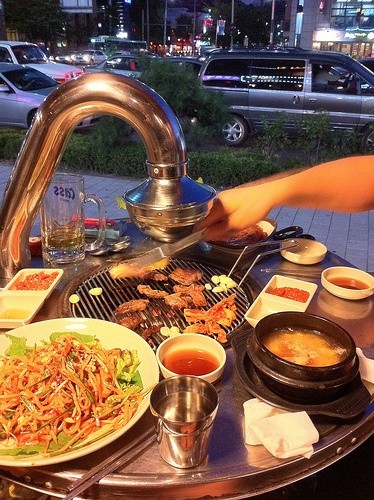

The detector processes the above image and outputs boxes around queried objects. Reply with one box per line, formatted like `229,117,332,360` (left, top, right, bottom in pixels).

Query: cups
150,375,220,468
39,173,106,264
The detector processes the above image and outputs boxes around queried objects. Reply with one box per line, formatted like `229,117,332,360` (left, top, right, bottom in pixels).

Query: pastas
0,335,132,448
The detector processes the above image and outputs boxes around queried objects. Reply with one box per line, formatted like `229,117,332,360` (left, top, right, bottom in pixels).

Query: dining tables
0,211,374,500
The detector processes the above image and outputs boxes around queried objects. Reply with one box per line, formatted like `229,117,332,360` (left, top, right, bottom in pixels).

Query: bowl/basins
280,239,328,265
246,311,360,401
318,288,372,320
155,333,226,383
321,266,374,301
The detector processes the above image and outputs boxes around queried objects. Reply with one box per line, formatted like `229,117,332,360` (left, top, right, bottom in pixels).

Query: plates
209,219,278,255
226,324,374,420
244,274,318,328
0,318,161,467
0,268,64,328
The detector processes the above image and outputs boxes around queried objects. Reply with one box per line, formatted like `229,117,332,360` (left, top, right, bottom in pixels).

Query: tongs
109,228,212,280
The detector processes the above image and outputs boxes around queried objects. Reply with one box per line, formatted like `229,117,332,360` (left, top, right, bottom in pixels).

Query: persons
193,154,374,500
315,64,352,84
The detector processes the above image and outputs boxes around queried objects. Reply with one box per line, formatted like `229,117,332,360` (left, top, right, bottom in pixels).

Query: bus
88,34,148,55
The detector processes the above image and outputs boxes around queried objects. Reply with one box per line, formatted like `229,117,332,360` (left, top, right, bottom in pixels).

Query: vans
198,46,374,154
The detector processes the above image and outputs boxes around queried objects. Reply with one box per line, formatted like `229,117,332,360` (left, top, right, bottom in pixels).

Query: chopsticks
61,377,222,499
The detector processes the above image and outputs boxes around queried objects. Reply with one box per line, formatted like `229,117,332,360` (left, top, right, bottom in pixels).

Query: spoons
85,235,133,257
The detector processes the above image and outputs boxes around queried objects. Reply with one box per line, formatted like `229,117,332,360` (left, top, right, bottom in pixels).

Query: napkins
240,395,319,459
357,347,374,399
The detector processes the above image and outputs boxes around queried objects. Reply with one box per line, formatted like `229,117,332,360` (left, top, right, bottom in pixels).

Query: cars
54,51,80,65
0,61,102,129
71,49,109,65
328,57,374,89
82,53,156,78
164,55,206,76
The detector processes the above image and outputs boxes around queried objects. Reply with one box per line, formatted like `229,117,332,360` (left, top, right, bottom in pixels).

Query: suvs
0,39,85,84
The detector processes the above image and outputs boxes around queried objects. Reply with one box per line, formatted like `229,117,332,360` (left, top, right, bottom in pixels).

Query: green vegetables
0,331,144,456
